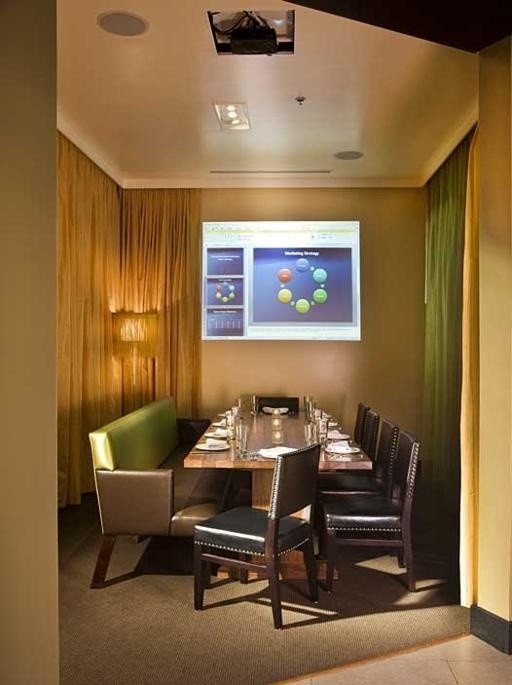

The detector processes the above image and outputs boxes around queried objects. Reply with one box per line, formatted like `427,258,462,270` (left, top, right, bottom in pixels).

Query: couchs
87,397,235,588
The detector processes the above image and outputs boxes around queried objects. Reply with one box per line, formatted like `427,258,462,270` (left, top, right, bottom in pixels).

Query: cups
227,399,251,460
304,395,327,447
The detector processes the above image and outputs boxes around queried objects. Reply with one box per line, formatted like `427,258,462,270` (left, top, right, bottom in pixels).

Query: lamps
112,312,157,416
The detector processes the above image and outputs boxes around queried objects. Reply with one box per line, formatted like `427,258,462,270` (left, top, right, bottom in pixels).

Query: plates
324,446,360,453
204,412,235,437
322,412,350,439
196,444,230,451
